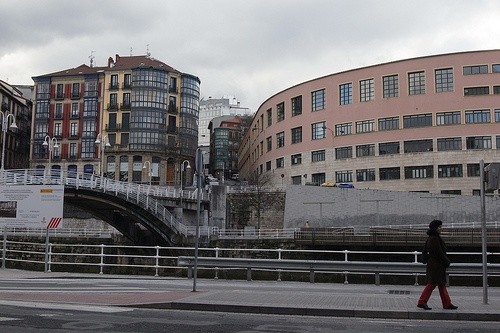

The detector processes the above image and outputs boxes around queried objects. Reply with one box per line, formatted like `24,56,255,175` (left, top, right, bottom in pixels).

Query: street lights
0,110,18,184
42,136,59,185
142,160,151,186
181,160,192,208
94,133,111,189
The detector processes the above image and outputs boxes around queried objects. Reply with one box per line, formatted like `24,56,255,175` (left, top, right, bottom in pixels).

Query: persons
304,220,310,228
417,220,459,309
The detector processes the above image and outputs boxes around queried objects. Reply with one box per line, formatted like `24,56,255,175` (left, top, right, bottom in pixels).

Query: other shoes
417,303,432,310
444,304,458,309
